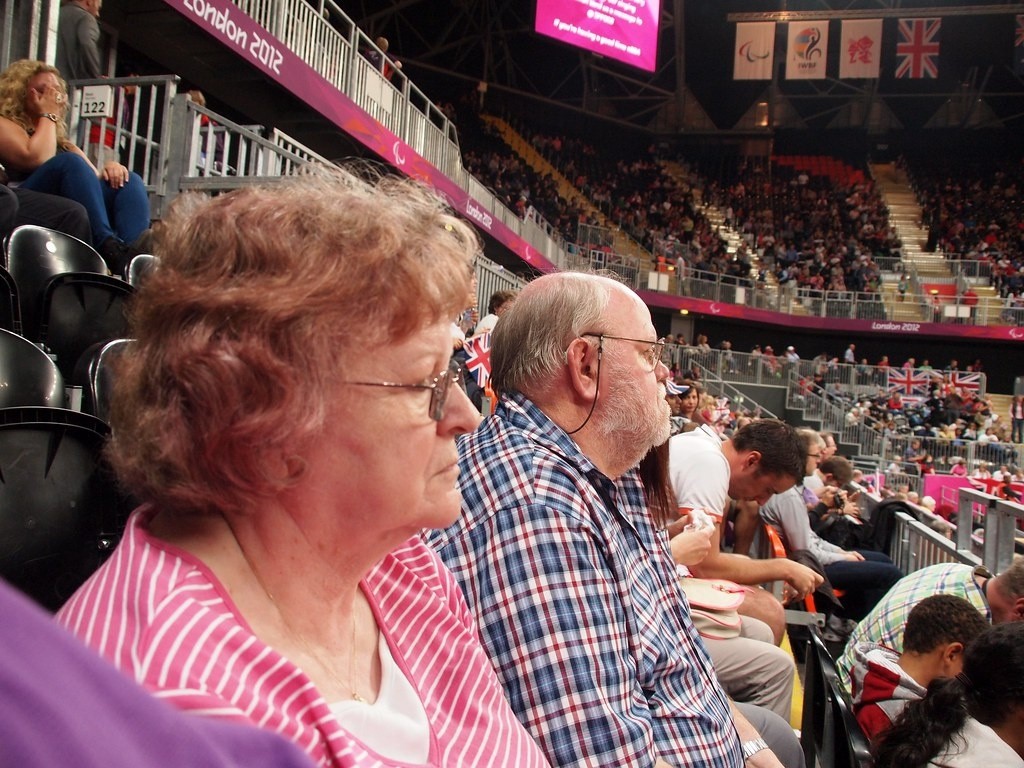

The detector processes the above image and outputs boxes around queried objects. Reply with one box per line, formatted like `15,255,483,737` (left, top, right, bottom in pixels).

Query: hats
665,379,691,395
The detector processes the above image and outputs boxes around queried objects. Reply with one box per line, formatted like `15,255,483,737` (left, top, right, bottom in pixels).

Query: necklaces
242,549,369,708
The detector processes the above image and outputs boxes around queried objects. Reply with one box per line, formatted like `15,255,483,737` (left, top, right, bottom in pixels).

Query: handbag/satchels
680,576,746,641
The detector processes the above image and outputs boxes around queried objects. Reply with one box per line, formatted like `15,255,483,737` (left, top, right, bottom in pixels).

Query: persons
85,62,139,175
0,60,151,279
364,36,403,75
309,94,1024,766
46,176,555,767
184,90,224,198
41,0,106,81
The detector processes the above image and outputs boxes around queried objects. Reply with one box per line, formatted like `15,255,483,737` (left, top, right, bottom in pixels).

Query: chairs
2,224,108,343
800,622,872,768
0,264,22,336
124,253,161,287
37,271,137,385
0,328,65,409
764,524,845,613
0,406,144,613
72,337,139,421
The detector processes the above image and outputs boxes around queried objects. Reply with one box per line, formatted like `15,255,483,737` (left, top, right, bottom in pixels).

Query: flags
461,330,495,390
897,18,941,80
733,20,775,81
786,20,829,79
839,18,884,80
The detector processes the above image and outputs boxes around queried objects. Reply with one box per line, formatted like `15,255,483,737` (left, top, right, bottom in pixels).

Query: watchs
39,112,58,123
739,736,769,764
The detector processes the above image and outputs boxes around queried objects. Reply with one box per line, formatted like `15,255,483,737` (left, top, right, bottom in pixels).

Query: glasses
579,332,666,371
328,358,466,420
806,453,820,460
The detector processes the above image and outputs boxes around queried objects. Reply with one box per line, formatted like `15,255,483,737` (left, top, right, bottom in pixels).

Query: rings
55,94,62,104
